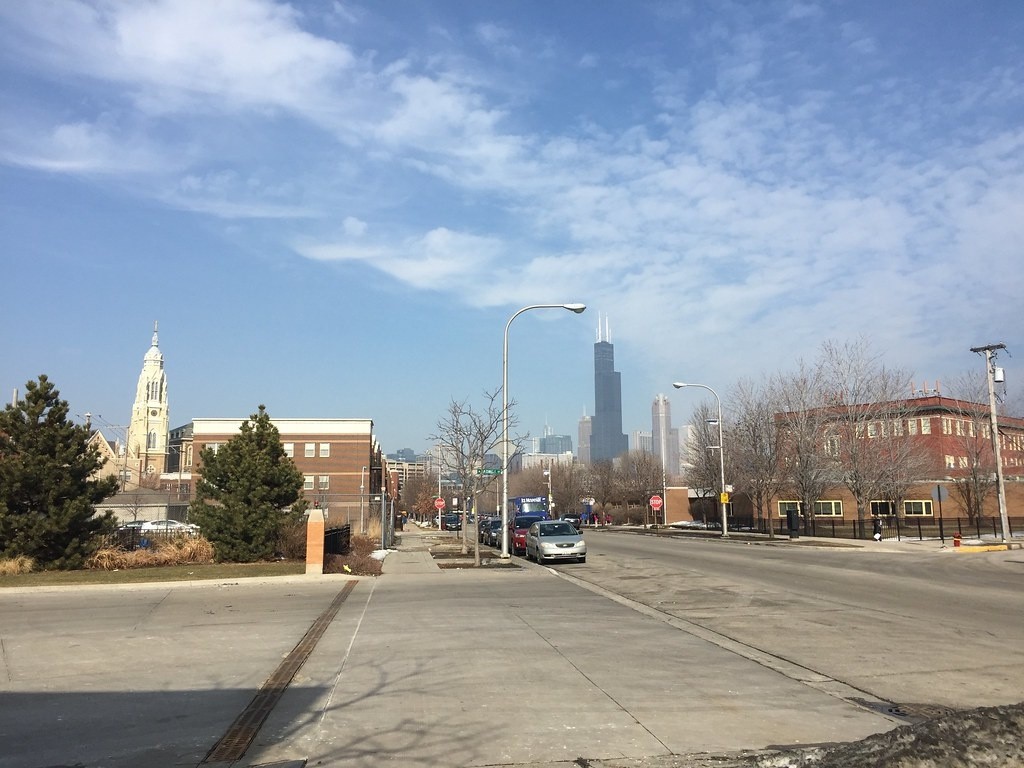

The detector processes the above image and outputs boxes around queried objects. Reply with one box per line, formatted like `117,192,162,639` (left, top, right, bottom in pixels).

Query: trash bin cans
395,515,403,532
442,521,446,530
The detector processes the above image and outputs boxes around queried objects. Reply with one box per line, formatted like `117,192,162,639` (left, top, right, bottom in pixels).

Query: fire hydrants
953,532,962,547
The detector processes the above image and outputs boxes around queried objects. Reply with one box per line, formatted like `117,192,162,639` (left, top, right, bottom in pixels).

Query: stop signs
435,498,445,508
650,496,663,509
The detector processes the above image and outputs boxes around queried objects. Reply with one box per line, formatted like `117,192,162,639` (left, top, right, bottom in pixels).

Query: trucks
507,496,552,521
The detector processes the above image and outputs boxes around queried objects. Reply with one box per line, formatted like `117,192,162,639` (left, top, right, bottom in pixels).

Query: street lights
527,453,553,518
498,303,588,561
672,382,730,538
437,430,472,532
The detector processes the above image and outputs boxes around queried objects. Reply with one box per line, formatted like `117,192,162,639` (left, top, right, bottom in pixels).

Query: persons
872,514,882,541
402,515,407,527
605,514,612,524
580,512,600,527
886,513,892,529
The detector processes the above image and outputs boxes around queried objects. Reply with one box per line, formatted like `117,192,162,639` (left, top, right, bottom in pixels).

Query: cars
525,519,587,566
559,513,582,530
509,515,550,557
477,513,510,550
432,513,470,531
